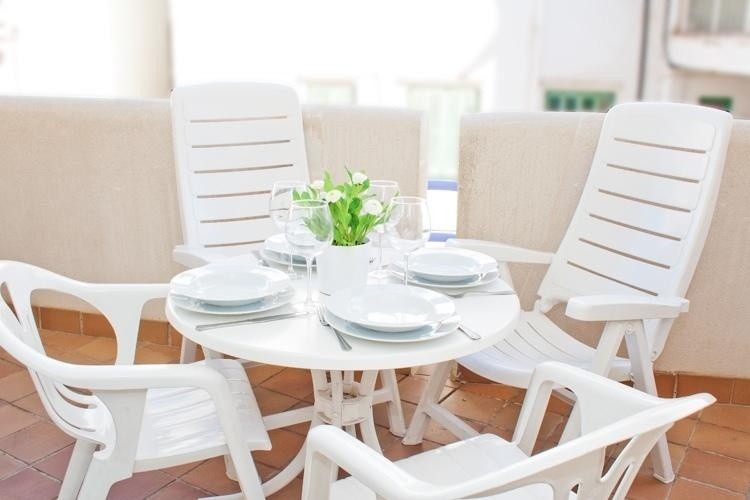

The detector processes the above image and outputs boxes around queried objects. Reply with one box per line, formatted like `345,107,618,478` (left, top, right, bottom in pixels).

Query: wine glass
271,181,308,260
368,182,404,248
288,200,333,314
385,197,430,282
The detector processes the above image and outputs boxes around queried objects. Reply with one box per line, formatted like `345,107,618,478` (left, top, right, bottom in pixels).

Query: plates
325,285,457,332
168,264,293,307
261,233,317,268
399,268,487,291
168,289,293,315
322,309,460,342
394,252,487,282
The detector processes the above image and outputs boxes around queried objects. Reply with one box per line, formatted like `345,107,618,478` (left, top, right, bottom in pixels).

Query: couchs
457,112,750,388
0,94,421,323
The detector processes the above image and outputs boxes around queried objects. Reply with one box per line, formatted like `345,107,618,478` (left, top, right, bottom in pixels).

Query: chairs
401,101,733,482
172,82,405,481
301,362,718,500
0,259,273,500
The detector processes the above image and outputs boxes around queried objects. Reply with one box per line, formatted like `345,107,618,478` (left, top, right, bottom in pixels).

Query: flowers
293,165,400,246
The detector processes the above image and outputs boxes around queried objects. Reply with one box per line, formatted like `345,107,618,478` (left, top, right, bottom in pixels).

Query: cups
427,181,459,242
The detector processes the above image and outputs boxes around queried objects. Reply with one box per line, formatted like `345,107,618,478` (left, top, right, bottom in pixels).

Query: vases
314,237,373,295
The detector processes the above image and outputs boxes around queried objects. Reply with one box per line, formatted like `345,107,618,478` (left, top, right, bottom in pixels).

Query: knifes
253,250,271,269
195,313,297,330
458,325,480,341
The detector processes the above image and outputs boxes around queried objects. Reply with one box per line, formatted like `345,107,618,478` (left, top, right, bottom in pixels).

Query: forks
319,315,352,351
444,291,516,296
